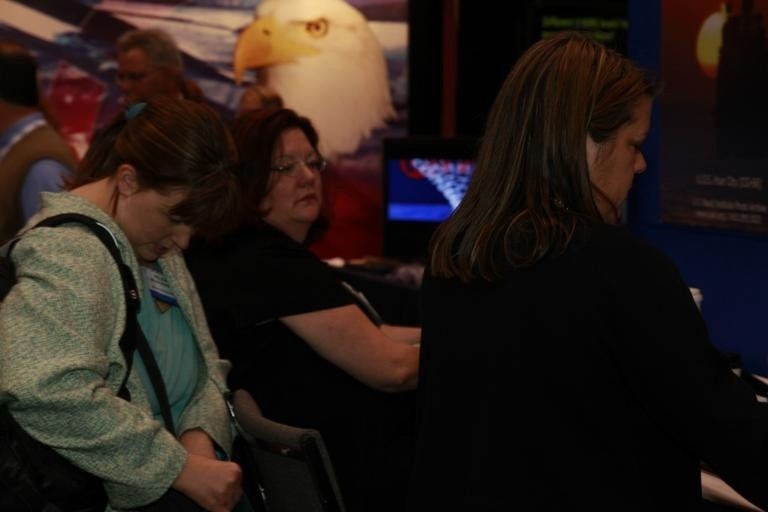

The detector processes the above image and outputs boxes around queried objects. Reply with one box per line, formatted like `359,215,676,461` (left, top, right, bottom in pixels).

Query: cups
270,151,327,174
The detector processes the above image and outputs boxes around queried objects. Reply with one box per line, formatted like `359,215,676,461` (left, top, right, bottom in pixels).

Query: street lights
229,406,344,512
0,385,132,512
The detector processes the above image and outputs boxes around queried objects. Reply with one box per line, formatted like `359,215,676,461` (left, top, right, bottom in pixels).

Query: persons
0,95,243,512
238,87,283,129
418,31,767,511
0,44,76,244
121,30,181,96
184,107,422,512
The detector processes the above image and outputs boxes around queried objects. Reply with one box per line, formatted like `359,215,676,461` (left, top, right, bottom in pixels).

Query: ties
379,137,483,264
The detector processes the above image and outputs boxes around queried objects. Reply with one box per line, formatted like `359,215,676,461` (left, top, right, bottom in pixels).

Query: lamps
233,389,349,510
